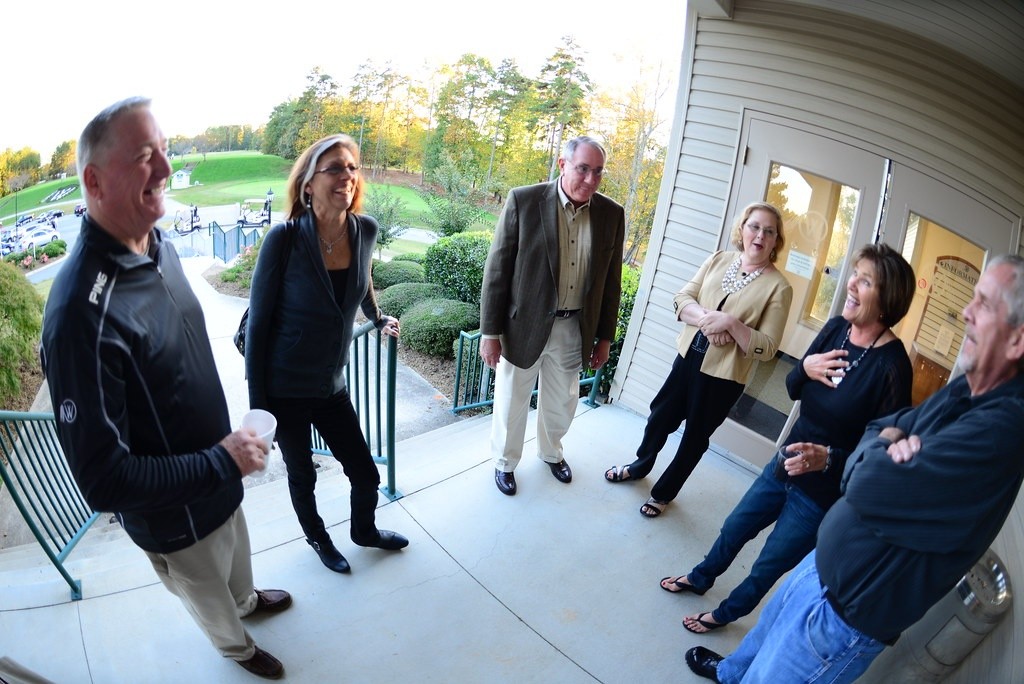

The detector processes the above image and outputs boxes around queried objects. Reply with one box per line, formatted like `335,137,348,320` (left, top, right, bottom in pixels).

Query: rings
824,370,828,376
803,460,810,468
715,340,718,344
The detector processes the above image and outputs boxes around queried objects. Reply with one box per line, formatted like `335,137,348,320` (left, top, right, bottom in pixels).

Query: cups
239,409,277,478
773,446,803,482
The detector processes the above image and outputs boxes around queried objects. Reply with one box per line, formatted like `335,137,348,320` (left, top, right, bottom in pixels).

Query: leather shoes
356,531,407,550
496,468,517,496
307,537,349,573
546,459,571,483
686,647,725,683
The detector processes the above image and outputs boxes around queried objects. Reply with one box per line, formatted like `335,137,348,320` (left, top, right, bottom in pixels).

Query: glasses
744,221,778,238
312,163,361,175
565,160,606,177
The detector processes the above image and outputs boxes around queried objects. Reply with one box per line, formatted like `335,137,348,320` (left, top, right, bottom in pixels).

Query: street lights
266,189,274,225
189,202,194,230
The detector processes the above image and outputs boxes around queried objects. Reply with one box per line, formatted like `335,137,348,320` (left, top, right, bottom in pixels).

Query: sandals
661,577,705,597
640,496,666,516
681,610,726,635
604,465,631,481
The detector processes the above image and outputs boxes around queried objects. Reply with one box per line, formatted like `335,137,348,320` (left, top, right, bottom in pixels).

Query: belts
556,309,580,318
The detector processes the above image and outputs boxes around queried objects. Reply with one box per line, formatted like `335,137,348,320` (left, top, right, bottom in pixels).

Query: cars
0,210,64,256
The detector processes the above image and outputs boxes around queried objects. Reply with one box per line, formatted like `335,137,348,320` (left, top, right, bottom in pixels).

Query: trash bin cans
849,546,1013,684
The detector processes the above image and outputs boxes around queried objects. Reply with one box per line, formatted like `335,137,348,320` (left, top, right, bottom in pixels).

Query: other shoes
253,587,291,611
239,648,283,679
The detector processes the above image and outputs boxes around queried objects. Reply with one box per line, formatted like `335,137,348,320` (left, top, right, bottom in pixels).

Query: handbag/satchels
234,221,293,356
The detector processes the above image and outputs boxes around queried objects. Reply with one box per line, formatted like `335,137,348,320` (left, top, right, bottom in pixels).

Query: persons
243,135,411,575
660,242,916,634
479,135,626,497
605,204,791,522
41,90,292,680
682,252,1024,684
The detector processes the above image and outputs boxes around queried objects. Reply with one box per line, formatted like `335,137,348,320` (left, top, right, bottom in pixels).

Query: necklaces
830,326,885,390
723,254,768,297
